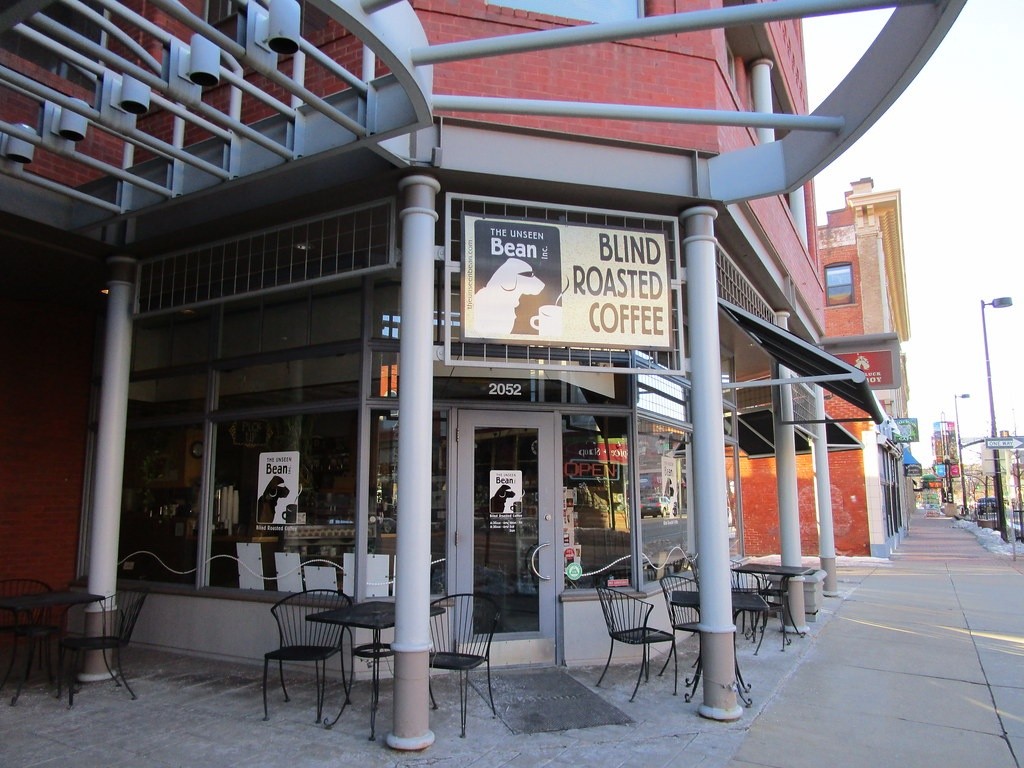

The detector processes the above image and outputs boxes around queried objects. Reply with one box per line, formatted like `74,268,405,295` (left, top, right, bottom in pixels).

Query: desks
732,563,811,656
305,601,446,742
671,591,770,708
0,591,106,707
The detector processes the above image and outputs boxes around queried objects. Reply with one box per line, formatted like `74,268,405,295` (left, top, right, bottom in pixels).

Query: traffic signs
984,436,1023,449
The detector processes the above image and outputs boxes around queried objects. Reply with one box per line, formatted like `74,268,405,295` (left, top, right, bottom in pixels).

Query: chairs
54,587,149,709
689,557,762,635
658,574,700,678
260,589,353,723
0,578,53,670
595,585,678,703
429,592,502,739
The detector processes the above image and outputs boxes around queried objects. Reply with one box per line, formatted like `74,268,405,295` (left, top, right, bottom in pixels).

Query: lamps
0,123,37,170
177,34,220,86
50,97,91,142
109,73,151,116
253,0,301,56
888,415,897,429
891,428,902,437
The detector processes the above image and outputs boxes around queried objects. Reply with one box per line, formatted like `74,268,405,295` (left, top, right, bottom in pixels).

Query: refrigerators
516,487,574,594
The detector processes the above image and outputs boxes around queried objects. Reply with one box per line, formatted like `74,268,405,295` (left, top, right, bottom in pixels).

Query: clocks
190,441,203,458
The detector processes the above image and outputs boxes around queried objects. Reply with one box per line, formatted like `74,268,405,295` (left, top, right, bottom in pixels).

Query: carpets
463,667,636,736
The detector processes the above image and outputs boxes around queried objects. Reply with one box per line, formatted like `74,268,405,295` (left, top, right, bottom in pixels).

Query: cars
974,496,997,516
641,495,678,518
1012,510,1023,542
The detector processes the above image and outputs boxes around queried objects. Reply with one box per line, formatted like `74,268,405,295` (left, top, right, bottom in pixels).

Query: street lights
955,394,971,518
980,297,1013,544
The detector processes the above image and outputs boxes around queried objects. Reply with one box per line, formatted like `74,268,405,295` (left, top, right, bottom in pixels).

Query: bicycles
525,540,541,576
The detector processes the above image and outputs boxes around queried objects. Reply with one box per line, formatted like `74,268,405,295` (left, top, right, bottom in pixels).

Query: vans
296,486,352,524
380,476,484,533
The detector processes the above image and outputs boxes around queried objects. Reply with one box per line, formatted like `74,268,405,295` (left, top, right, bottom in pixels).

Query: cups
282,504,297,523
510,502,521,512
